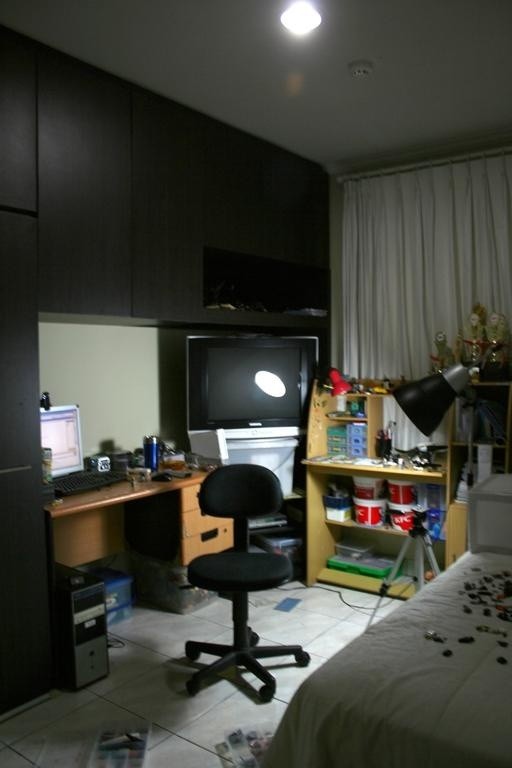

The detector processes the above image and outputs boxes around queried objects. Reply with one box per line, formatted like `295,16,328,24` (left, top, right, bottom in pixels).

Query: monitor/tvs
40,404,85,478
184,333,319,431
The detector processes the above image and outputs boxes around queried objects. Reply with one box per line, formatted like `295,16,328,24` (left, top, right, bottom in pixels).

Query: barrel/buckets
386,479,419,505
386,495,419,535
351,475,386,501
352,496,386,527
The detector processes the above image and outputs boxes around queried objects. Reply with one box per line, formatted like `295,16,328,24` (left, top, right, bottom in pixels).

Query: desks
2,467,233,696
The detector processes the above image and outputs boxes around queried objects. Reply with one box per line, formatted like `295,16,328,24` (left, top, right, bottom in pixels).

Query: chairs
184,463,311,702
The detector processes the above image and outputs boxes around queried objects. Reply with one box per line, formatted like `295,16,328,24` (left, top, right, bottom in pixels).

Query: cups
376,439,392,457
144,435,161,471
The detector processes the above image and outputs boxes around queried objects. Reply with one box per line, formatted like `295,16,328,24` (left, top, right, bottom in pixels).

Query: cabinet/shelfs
302,377,511,601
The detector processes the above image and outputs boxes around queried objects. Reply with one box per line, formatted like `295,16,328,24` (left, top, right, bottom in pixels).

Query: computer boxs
50,558,112,691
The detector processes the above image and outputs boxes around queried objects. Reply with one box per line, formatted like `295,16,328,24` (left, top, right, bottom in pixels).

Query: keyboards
51,469,127,497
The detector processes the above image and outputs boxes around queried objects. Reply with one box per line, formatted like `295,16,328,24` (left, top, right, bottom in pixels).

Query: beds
263,553,511,765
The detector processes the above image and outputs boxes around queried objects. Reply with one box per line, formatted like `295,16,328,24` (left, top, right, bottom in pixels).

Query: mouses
150,471,173,483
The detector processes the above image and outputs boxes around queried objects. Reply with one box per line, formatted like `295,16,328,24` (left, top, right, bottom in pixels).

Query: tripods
364,504,440,632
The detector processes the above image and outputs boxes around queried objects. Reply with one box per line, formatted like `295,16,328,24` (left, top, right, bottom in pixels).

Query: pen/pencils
376,428,391,440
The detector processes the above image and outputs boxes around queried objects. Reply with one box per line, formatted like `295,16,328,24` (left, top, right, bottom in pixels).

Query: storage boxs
326,536,405,580
85,535,305,629
